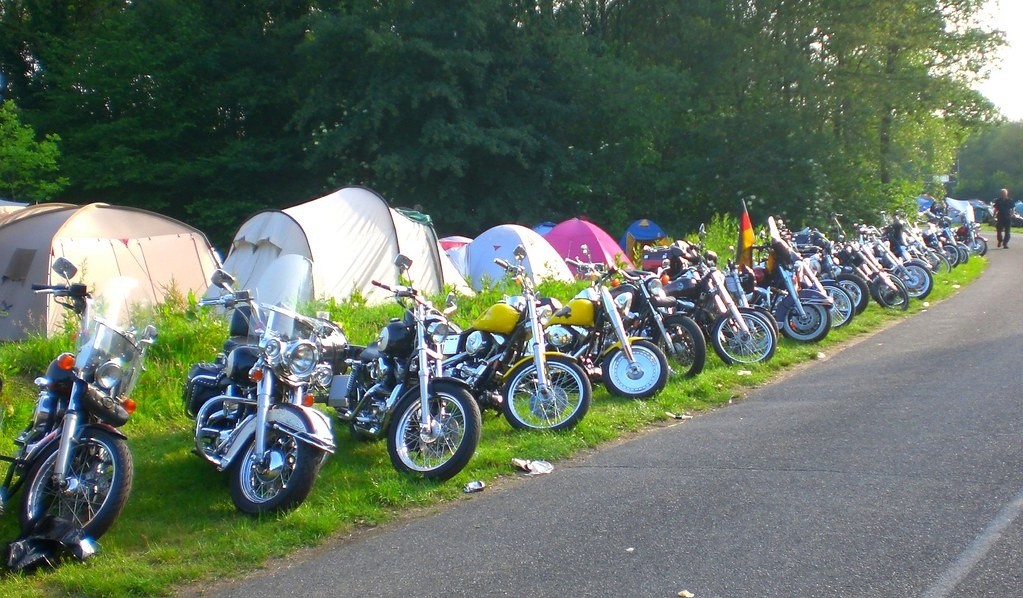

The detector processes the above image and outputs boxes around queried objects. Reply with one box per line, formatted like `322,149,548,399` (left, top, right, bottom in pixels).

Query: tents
200,186,671,314
0,197,221,347
915,196,1023,225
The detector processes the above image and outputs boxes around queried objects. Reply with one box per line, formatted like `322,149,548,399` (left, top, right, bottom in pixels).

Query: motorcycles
332,255,483,487
519,196,990,403
0,257,159,548
182,269,351,522
411,244,593,436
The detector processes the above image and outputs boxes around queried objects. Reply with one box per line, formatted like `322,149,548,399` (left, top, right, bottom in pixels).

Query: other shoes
1003,243,1008,249
998,240,1002,247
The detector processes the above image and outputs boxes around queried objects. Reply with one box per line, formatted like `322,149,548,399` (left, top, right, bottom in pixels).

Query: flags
735,199,756,272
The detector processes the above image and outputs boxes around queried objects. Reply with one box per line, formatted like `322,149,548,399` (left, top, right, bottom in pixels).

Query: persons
990,188,1014,249
